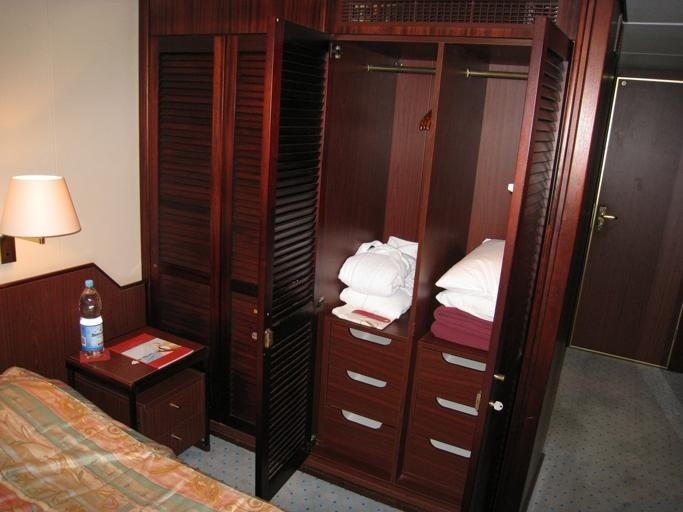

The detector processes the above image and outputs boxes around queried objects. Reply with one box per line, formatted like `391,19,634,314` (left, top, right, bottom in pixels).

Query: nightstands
65,326,211,457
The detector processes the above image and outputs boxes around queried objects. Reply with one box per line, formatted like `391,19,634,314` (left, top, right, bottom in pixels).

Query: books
107,333,193,370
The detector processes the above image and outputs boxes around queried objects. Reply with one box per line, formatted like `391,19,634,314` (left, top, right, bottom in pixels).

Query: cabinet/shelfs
139,0,321,455
254,16,574,512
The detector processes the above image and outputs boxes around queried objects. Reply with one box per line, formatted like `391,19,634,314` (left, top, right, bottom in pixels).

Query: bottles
77,279,106,358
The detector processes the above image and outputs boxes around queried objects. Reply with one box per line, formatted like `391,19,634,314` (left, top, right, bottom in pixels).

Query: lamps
0,174,81,264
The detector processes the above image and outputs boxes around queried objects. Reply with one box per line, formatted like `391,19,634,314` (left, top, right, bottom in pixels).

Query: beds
0,366,283,512
